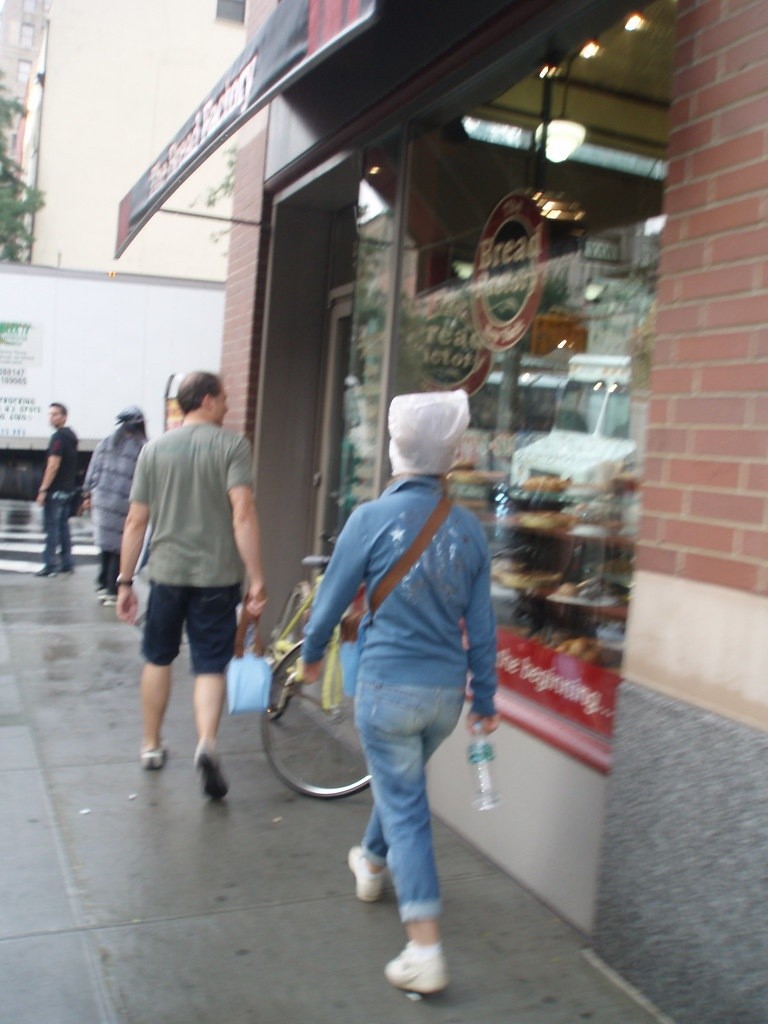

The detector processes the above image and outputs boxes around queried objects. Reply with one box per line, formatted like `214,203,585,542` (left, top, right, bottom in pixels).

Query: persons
117,372,267,798
300,389,502,996
81,404,148,609
36,403,81,578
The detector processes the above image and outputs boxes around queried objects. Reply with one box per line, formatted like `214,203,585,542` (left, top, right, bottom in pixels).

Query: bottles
467,724,498,809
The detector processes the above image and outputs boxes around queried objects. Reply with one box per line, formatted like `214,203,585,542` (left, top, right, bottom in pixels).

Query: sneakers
194,741,229,800
384,941,450,994
96,585,117,609
347,845,383,903
35,563,75,579
140,747,168,770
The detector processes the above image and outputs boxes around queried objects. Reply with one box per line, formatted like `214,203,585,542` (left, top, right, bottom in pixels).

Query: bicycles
258,531,379,797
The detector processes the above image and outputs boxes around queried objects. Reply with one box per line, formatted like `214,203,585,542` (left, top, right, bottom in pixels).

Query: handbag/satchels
337,608,371,644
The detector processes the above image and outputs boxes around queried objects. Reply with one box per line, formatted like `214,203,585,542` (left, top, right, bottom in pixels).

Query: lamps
533,54,587,163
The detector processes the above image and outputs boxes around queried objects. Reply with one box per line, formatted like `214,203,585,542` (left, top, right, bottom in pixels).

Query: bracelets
116,576,133,587
38,488,47,492
82,495,90,500
